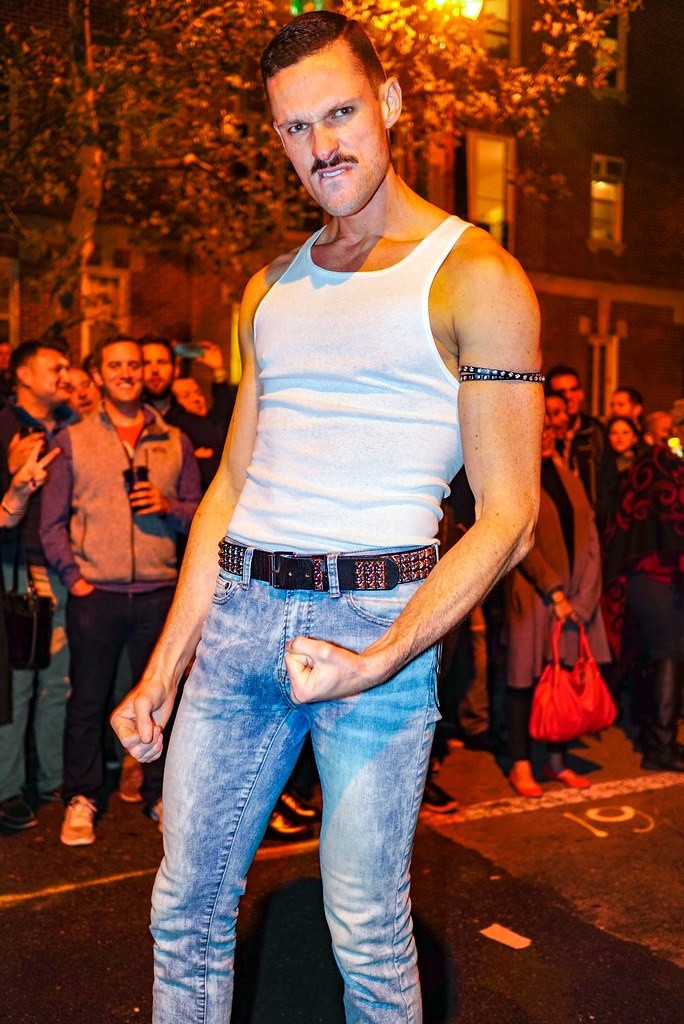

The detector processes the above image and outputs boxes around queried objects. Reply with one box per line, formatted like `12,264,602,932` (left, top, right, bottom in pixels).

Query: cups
122,465,150,514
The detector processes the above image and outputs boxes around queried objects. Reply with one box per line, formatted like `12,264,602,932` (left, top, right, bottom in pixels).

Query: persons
108,11,542,1024
418,366,684,811
0,335,325,846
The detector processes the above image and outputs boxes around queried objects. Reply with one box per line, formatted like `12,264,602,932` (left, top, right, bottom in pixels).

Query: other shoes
555,769,591,789
421,780,460,813
259,811,312,841
273,790,322,821
639,755,684,773
507,761,544,798
0,795,38,829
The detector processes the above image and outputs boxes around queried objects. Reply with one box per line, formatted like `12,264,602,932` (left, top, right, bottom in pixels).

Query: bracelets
2,502,28,515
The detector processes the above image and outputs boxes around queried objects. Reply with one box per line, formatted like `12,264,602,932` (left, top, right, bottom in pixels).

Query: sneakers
60,796,97,846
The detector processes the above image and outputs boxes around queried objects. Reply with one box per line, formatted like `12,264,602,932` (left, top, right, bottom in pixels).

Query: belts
218,537,436,592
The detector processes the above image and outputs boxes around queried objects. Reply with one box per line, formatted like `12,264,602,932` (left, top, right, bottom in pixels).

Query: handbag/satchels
0,530,53,670
529,614,618,742
